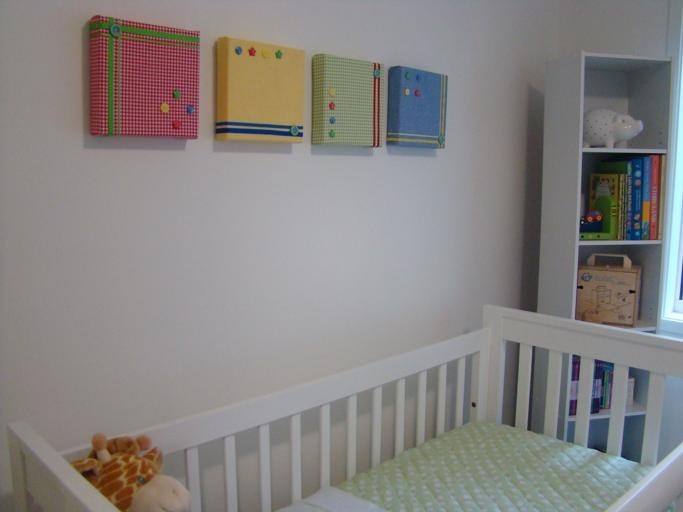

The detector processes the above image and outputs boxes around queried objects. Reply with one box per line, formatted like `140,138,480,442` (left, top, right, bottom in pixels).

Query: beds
6,305,682,512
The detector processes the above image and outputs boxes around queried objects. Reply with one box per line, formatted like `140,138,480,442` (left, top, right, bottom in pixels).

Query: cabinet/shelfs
530,49,675,460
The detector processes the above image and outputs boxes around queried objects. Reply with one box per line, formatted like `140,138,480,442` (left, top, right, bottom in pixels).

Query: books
588,153,669,242
566,355,636,417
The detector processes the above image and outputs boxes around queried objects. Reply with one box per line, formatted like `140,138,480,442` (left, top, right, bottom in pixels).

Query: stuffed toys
71,430,192,512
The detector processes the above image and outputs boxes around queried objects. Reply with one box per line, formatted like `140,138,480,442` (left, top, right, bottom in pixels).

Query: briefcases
574,253,642,328
